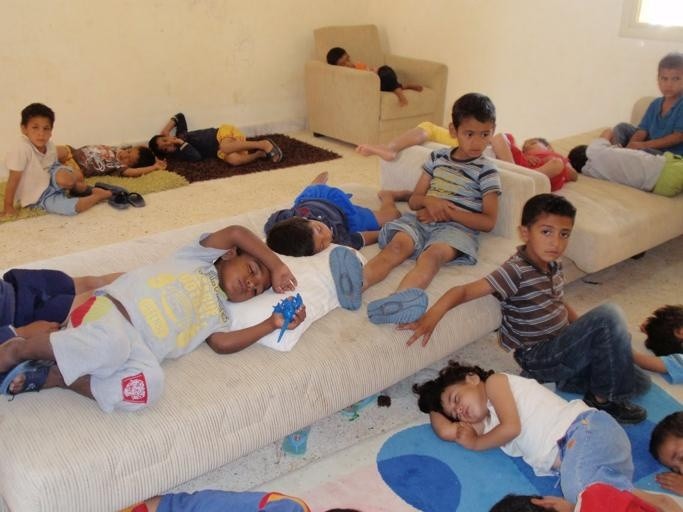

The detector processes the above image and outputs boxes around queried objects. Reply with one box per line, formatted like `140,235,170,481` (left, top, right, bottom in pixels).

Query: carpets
0,143,188,222
243,363,682,512
156,133,341,184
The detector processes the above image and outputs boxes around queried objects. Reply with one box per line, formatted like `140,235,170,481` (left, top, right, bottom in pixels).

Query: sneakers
584,391,647,423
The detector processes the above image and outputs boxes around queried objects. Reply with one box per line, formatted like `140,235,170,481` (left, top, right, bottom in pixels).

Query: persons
326,46,424,108
489,482,660,511
148,112,283,168
410,359,681,511
355,120,459,162
3,102,146,216
1,225,307,416
53,145,167,177
569,126,682,199
610,51,683,158
648,411,683,496
489,132,578,193
264,171,414,257
395,192,649,424
327,93,503,326
628,305,683,385
120,489,358,512
0,268,126,339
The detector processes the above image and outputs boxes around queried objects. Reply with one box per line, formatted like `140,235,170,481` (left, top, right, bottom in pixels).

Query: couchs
419,94,681,274
0,144,535,511
303,24,447,149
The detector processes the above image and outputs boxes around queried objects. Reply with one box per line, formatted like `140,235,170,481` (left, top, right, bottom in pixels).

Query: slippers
71,183,145,208
330,247,362,309
1,360,56,394
341,395,377,416
263,137,284,164
368,289,429,324
283,426,310,455
